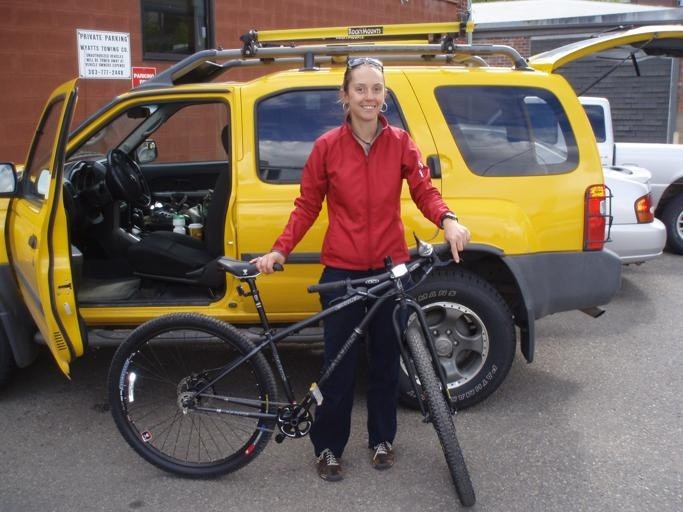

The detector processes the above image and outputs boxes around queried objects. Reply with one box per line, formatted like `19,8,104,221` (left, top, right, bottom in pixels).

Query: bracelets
441,213,458,226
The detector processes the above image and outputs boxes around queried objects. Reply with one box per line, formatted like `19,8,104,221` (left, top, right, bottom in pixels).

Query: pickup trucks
484,96,683,257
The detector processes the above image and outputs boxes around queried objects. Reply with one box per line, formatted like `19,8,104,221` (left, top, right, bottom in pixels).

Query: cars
451,122,668,264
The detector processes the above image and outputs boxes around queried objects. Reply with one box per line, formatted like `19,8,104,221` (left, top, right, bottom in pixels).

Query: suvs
0,13,683,416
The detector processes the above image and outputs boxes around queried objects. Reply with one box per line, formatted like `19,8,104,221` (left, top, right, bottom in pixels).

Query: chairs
125,125,232,296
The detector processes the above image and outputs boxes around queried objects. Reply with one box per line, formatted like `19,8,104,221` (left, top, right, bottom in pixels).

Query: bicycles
108,232,477,507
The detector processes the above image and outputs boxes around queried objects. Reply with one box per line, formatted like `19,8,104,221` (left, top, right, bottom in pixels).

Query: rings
256,255,263,262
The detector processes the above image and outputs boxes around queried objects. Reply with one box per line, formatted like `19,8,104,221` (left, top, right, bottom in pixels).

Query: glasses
348,57,382,68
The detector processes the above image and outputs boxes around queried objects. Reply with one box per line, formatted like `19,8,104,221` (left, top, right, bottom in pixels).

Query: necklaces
351,117,380,153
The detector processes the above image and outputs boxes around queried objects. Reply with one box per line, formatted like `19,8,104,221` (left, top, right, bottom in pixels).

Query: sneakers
372,441,394,469
317,448,342,481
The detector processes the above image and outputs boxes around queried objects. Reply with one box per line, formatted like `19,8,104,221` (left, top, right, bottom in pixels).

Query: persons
250,56,470,482
